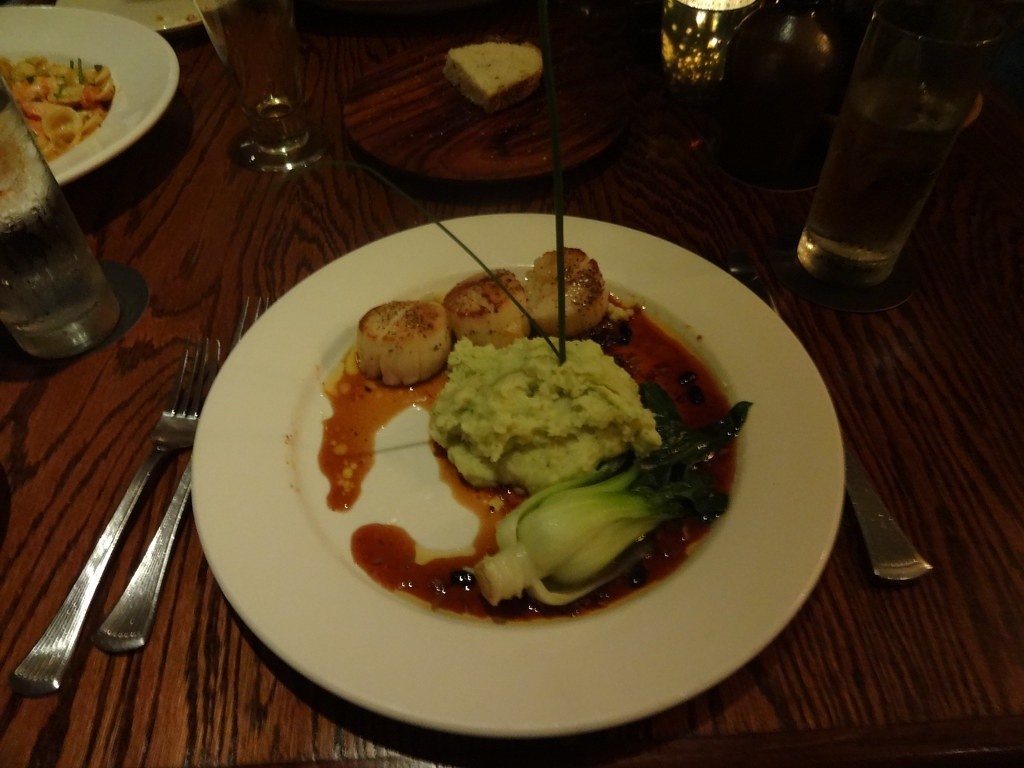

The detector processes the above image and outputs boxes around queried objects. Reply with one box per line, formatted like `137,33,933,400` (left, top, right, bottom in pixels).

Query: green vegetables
480,380,757,628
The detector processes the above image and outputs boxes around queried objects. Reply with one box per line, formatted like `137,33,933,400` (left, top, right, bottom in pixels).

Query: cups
797,2,1008,294
661,0,762,87
0,80,118,359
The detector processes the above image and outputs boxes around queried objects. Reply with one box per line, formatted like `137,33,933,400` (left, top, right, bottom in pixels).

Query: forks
15,296,272,694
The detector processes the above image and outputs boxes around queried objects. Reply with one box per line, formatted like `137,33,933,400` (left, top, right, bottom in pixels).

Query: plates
0,0,229,217
189,214,844,736
345,34,628,180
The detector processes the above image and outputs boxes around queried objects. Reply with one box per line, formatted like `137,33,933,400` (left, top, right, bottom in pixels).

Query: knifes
722,245,933,583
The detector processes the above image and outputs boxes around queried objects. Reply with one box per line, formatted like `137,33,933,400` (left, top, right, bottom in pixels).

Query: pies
355,248,606,385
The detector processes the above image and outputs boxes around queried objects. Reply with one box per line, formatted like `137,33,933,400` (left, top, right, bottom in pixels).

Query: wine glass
217,0,326,173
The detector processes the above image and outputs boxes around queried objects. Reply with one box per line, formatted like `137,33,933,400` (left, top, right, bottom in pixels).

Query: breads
442,40,543,111
